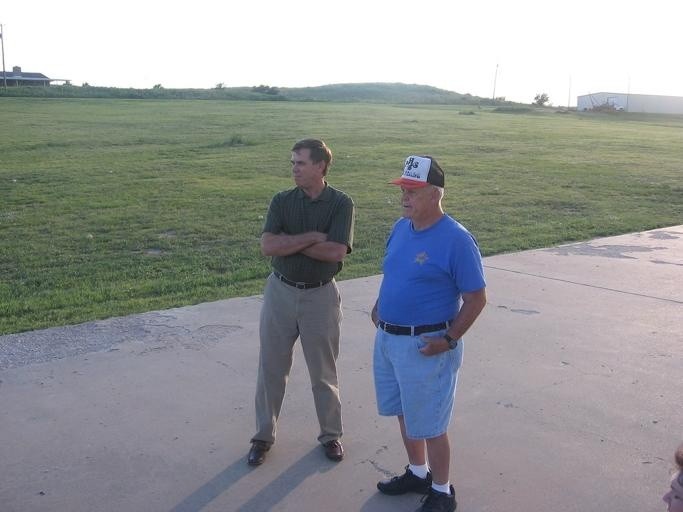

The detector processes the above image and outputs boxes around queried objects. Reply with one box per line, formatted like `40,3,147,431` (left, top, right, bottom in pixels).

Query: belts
271,270,333,290
375,318,454,335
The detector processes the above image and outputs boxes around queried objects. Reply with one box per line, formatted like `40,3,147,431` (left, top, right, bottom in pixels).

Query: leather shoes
323,440,345,462
246,439,272,466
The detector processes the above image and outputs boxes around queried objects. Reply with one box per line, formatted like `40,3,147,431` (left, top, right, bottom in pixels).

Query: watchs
444,334,457,349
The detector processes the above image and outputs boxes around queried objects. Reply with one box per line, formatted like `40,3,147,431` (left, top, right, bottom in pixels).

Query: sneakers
376,464,433,495
415,484,458,512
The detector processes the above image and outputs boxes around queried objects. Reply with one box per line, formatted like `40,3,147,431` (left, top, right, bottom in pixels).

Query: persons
371,155,487,512
248,138,355,466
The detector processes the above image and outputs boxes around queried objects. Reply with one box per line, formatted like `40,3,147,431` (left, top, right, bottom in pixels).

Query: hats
387,155,446,190
